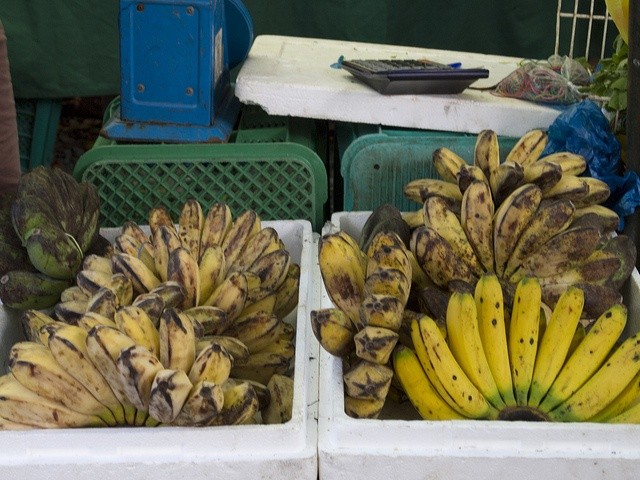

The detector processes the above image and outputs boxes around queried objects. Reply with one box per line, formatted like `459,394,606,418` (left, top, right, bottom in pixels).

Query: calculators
340,60,489,94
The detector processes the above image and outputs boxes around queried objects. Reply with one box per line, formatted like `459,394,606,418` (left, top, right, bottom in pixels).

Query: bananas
312,205,414,419
0,165,299,429
404,128,640,317
394,275,638,422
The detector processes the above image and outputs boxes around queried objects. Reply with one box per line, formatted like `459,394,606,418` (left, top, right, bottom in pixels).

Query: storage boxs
320,211,640,480
0,219,319,480
569,82,619,131
